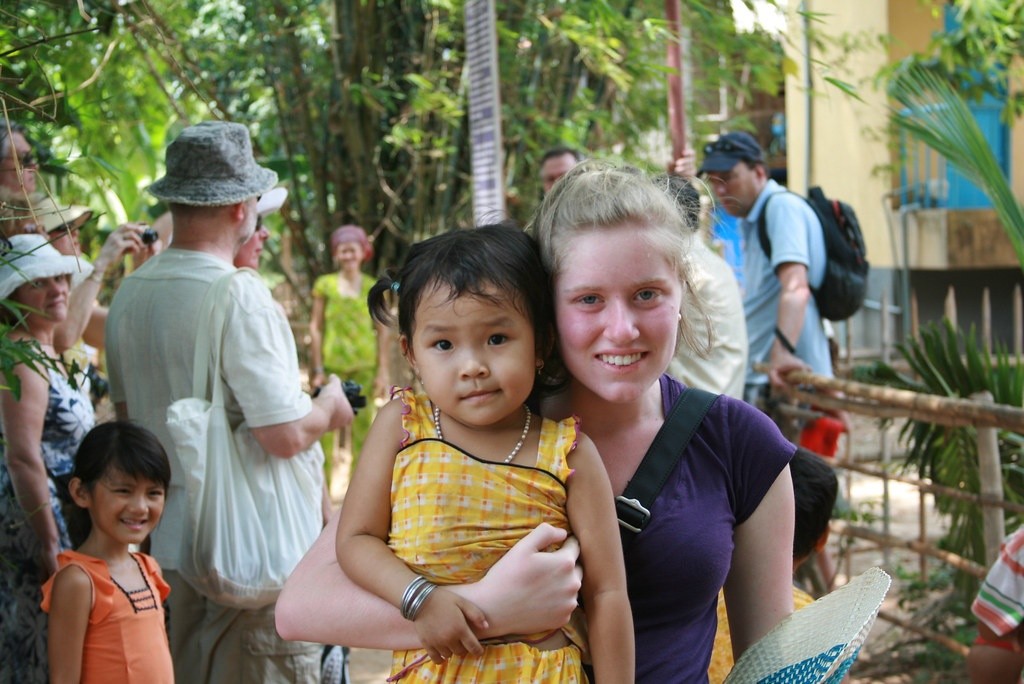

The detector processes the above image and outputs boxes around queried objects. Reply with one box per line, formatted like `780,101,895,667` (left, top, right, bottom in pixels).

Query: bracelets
90,269,101,282
400,575,438,622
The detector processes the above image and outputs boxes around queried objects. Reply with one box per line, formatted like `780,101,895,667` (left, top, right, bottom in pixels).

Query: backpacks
755,185,868,319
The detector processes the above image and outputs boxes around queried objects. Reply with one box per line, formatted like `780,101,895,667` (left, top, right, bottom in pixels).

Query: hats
0,232,97,305
29,196,95,235
717,567,891,684
695,132,765,180
148,124,282,208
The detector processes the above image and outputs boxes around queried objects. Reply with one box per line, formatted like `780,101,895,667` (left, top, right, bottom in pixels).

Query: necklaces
435,403,531,464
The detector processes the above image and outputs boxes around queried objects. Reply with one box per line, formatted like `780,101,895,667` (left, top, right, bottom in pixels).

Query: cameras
135,223,158,243
312,380,366,415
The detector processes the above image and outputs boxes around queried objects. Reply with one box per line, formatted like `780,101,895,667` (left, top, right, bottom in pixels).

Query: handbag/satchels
165,267,325,613
52,472,92,550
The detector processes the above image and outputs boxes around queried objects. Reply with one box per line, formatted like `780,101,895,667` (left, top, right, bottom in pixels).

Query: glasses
52,222,82,233
0,154,39,169
701,141,758,156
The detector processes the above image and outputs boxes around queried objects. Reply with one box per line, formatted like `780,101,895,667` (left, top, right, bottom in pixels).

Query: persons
308,226,393,525
539,133,1024,684
335,223,636,684
0,119,354,684
275,159,798,684
